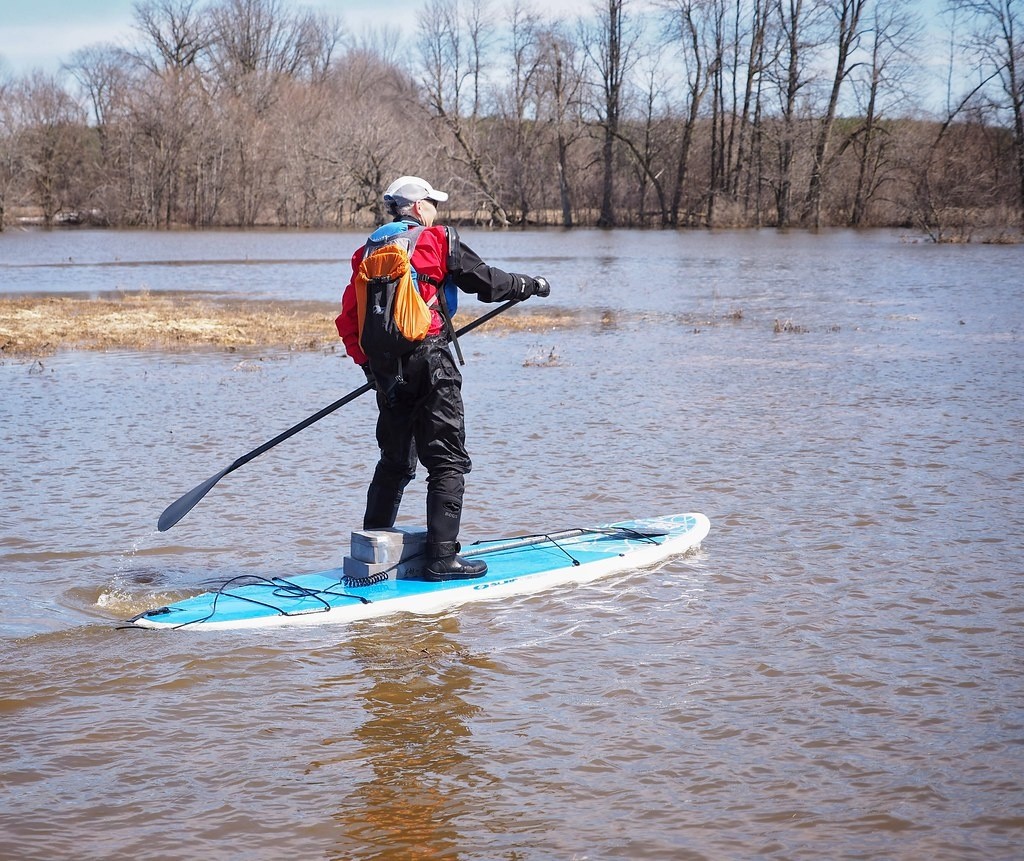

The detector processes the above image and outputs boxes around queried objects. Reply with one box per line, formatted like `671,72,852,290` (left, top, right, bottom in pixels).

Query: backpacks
354,226,431,359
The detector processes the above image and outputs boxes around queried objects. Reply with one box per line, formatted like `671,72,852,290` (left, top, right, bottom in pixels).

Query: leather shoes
423,541,488,580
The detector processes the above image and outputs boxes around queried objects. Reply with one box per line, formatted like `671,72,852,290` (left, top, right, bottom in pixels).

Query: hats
384,176,449,214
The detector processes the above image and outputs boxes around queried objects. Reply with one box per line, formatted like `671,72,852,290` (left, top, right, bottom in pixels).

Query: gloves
359,359,377,390
532,276,550,297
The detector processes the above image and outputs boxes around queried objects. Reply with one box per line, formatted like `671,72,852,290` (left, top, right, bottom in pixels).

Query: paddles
158,294,535,532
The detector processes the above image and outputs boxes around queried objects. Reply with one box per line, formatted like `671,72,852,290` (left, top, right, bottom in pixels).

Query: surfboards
132,510,712,633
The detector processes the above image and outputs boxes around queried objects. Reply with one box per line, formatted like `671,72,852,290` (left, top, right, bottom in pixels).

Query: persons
335,171,551,583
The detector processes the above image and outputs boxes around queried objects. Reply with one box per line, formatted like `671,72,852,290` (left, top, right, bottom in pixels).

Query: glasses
421,199,438,208
